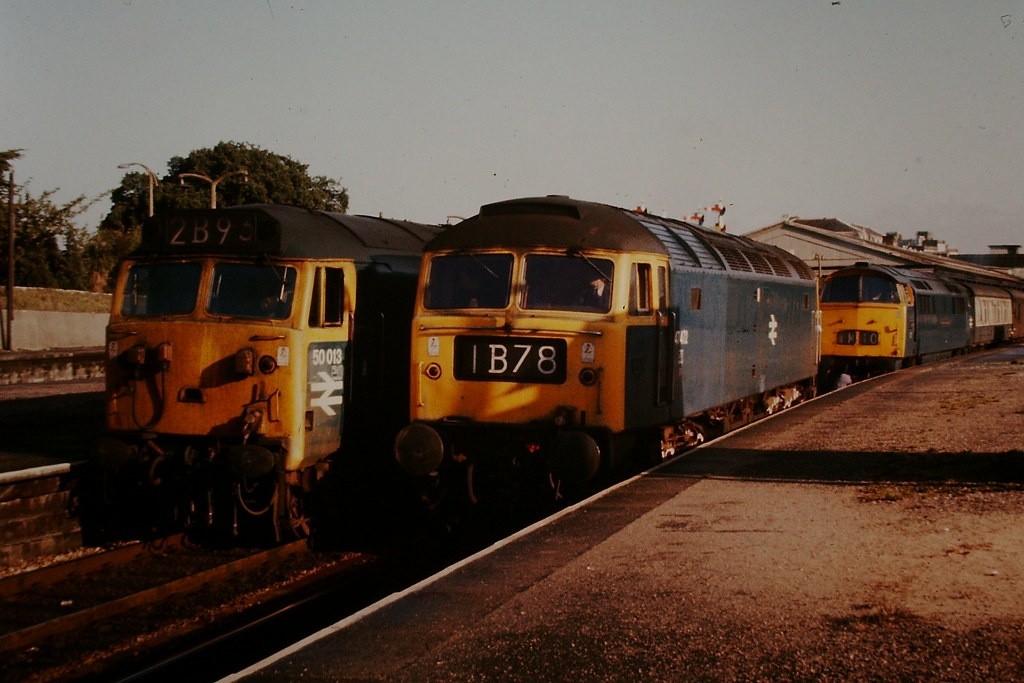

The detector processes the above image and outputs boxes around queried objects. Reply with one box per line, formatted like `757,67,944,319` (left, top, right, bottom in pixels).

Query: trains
391,194,818,527
99,204,449,527
820,258,1023,393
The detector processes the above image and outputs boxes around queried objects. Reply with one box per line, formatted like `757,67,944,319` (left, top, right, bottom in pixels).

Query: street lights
118,161,158,219
177,170,247,210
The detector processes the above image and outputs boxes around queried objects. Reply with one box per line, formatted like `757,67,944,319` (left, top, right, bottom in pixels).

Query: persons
584,271,611,313
